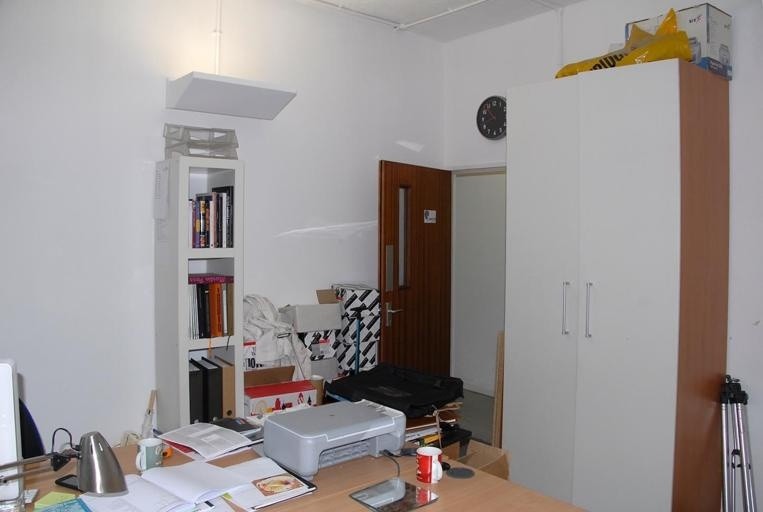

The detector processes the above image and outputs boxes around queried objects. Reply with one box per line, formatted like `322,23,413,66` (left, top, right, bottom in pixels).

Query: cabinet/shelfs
501,57,730,512
156,156,244,433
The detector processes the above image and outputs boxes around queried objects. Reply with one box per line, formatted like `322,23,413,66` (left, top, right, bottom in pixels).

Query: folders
186,356,232,424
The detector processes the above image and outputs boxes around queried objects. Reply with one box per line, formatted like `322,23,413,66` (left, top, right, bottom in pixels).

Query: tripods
719,374,758,512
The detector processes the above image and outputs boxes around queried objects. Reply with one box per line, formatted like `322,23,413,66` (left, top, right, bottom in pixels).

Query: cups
134,437,162,471
415,446,443,484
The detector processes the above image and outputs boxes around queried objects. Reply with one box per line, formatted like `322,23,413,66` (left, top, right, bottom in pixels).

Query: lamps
0,428,129,498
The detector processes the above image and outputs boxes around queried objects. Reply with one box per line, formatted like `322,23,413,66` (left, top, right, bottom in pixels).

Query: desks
1,443,589,512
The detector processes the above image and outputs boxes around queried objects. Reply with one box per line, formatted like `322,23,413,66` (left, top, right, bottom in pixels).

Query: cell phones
55,473,79,490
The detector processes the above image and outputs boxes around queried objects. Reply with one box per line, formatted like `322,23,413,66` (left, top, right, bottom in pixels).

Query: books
401,426,444,446
188,186,234,248
188,272,234,340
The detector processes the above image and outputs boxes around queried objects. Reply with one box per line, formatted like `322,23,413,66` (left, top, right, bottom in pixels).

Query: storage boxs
624,2,733,81
244,286,381,417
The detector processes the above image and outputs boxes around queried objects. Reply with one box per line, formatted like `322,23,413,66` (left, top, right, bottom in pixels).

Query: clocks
476,95,506,140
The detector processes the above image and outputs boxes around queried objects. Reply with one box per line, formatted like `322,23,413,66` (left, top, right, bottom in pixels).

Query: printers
263,398,407,480
351,477,407,508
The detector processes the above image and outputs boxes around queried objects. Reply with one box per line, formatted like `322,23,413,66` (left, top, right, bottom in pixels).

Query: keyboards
21,488,39,504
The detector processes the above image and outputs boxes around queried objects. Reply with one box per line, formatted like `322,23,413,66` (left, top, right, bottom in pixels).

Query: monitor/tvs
0,358,24,503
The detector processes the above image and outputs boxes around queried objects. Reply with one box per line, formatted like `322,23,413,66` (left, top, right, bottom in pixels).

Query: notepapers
34,490,74,508
